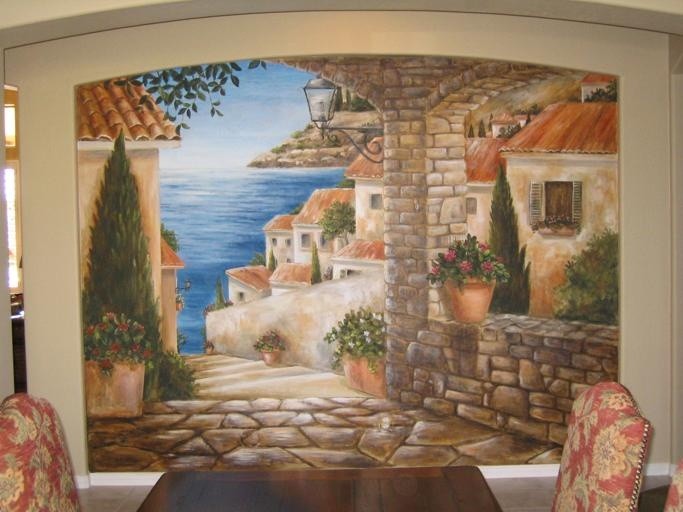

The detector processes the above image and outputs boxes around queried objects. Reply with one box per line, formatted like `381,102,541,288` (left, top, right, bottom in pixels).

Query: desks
137,466,501,510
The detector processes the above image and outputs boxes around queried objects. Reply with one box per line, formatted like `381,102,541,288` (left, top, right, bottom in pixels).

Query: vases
83,359,148,418
261,348,280,364
340,351,385,398
444,275,496,325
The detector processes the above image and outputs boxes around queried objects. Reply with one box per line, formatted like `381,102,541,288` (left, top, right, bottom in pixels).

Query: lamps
301,72,385,166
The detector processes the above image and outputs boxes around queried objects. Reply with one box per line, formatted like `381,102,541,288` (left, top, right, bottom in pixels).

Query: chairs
546,377,654,511
0,389,83,512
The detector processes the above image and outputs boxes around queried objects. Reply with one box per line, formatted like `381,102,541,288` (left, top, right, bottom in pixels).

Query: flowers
323,305,385,375
82,310,156,381
427,231,512,294
251,328,286,353
